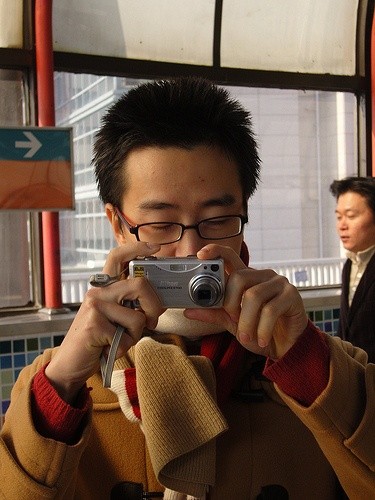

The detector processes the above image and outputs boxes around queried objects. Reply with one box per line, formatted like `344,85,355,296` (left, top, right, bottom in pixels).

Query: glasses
115,195,249,247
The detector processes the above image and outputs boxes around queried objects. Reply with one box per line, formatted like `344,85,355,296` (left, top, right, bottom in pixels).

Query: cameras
128,254,224,310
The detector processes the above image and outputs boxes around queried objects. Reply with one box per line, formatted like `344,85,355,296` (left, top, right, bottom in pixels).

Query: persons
327,176,375,366
0,77,375,500
